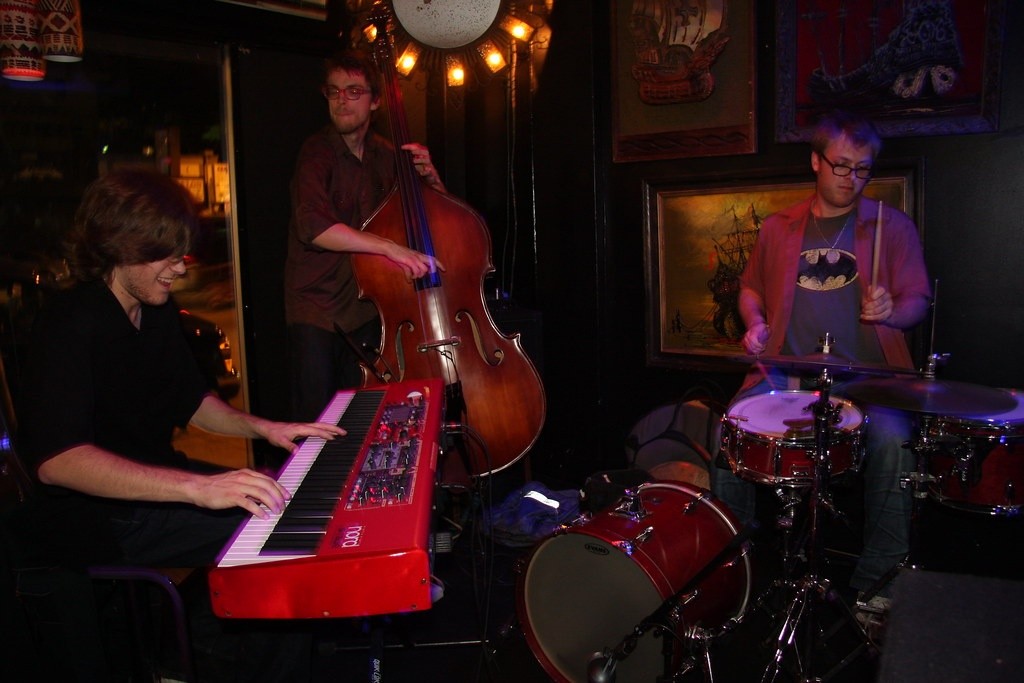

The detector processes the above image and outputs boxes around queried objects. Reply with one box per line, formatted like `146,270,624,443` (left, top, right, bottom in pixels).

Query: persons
286,58,451,420
16,165,347,683
713,114,931,629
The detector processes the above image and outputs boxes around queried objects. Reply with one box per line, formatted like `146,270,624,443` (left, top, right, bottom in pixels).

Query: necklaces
814,206,852,263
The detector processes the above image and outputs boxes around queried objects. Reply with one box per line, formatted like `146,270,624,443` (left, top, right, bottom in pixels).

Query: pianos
206,377,497,683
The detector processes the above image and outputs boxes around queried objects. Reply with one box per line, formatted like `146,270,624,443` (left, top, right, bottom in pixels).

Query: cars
0,258,238,401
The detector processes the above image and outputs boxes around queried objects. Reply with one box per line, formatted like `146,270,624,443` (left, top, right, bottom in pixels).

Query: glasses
322,85,371,100
818,150,874,179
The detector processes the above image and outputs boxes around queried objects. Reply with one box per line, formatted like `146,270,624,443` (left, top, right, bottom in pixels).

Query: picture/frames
773,0,1006,144
640,154,923,374
610,0,759,163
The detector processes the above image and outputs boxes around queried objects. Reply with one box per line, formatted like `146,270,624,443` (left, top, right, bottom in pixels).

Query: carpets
875,570,1024,683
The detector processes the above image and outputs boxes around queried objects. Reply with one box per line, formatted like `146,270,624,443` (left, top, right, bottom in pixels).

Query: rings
422,164,426,171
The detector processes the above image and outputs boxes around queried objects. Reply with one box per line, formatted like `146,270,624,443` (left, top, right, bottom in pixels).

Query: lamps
358,0,536,90
0,0,83,82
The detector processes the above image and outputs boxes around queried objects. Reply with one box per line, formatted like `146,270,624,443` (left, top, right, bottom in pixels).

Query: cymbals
740,352,923,375
839,378,1019,416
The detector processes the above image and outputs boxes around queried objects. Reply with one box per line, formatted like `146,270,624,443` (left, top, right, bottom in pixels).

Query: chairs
0,304,190,683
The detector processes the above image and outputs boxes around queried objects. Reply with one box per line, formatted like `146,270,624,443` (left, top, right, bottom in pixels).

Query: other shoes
855,591,890,655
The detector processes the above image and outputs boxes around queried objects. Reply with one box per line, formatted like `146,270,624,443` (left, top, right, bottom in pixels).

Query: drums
918,387,1024,513
724,390,867,487
511,479,757,683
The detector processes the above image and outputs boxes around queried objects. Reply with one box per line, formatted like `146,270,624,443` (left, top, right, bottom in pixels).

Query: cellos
351,0,546,478
